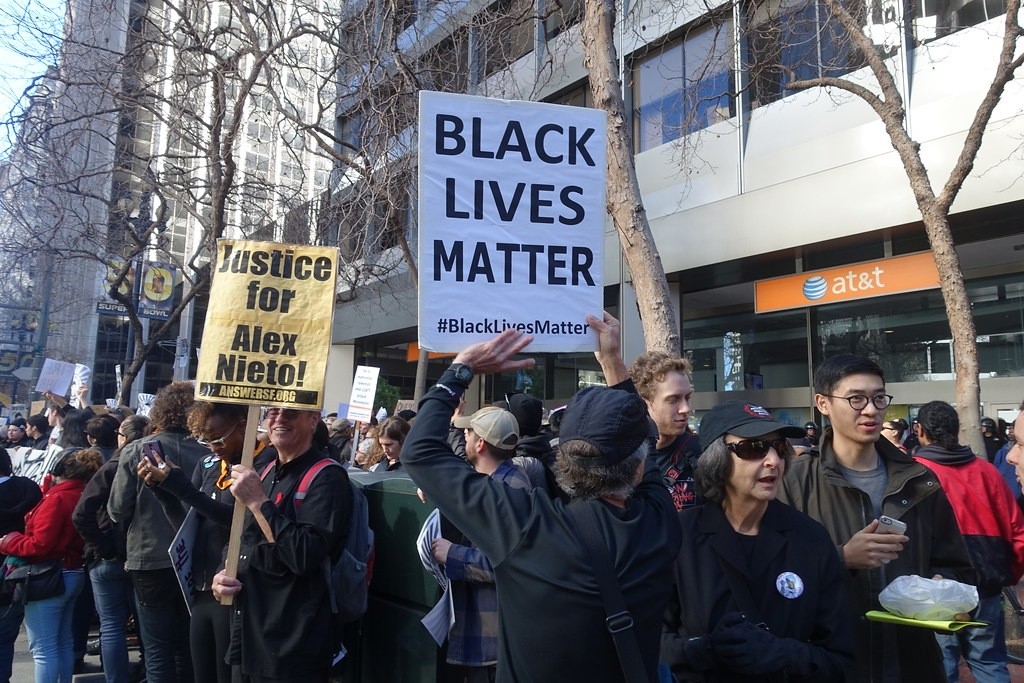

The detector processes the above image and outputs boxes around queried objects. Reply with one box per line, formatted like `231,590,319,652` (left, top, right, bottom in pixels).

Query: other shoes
85,637,100,655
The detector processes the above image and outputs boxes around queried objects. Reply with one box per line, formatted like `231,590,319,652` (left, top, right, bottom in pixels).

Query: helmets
911,417,918,429
803,422,817,431
980,418,996,432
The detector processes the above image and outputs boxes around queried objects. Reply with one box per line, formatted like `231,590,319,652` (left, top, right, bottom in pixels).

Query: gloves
688,612,810,683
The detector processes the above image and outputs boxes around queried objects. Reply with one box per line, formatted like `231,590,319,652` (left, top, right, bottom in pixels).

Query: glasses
116,408,125,416
267,408,310,421
723,437,789,459
197,419,247,448
881,427,895,432
115,429,125,437
821,393,893,410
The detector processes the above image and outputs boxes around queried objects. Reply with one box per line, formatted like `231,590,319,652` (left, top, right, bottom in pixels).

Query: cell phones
142,440,165,466
874,516,907,565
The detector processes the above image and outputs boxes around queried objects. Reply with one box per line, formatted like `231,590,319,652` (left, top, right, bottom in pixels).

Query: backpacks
258,458,373,627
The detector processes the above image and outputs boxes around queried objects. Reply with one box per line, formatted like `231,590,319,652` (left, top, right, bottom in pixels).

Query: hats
398,410,417,422
10,417,27,432
559,386,659,466
331,419,353,432
510,394,543,437
697,399,807,454
453,407,519,449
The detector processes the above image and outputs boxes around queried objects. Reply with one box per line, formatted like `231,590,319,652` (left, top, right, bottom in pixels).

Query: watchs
448,363,474,383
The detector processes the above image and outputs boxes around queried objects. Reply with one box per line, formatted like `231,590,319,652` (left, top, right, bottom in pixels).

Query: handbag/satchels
6,560,66,601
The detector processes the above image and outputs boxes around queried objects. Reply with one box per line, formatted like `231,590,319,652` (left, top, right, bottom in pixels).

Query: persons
913,400,1024,683
628,351,699,683
107,380,213,683
449,393,566,499
72,416,152,683
319,409,415,472
0,448,43,683
881,418,921,456
659,399,858,683
982,404,1024,508
776,354,972,683
788,420,818,460
416,405,533,683
0,383,141,672
137,408,275,683
400,312,683,683
210,406,354,683
0,447,104,683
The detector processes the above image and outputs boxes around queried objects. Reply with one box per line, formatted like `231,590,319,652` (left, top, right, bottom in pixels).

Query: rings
158,463,166,468
